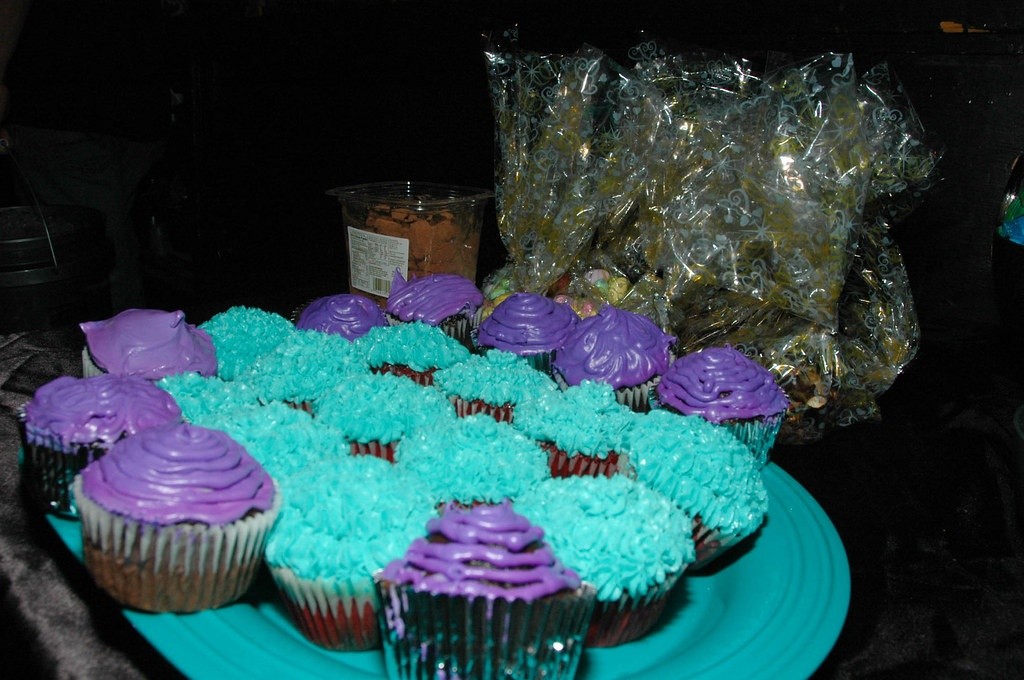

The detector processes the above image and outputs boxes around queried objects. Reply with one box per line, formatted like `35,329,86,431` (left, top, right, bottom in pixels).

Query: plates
13,445,851,680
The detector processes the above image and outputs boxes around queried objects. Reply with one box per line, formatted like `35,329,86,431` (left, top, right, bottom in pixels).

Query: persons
0,1,30,149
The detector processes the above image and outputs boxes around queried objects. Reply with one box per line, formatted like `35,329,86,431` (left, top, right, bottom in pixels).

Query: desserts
19,274,791,680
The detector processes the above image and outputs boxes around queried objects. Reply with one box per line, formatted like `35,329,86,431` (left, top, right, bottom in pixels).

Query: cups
324,179,496,311
